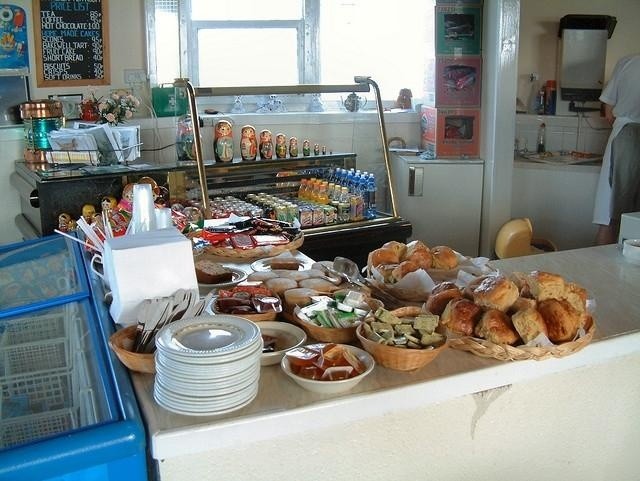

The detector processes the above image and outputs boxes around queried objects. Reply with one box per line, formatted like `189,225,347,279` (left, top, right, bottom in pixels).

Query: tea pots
340,93,368,113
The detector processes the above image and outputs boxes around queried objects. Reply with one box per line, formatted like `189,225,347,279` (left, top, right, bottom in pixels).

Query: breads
195,258,342,306
372,239,588,345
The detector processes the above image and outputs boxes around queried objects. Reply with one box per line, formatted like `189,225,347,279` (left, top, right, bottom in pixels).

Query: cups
129,183,173,234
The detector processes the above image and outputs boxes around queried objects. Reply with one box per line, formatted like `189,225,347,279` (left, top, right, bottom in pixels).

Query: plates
198,258,312,288
152,314,265,418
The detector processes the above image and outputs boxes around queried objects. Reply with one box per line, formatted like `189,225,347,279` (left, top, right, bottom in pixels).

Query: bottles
298,165,378,222
537,80,556,154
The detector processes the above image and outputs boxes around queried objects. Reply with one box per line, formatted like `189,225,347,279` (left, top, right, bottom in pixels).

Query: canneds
187,193,300,229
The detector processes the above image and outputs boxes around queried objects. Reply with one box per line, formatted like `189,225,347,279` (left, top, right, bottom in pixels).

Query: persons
591,54,640,246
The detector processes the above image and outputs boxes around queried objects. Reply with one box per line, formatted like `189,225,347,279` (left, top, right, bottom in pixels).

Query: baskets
370,247,597,361
293,297,384,343
1,313,77,447
355,306,447,372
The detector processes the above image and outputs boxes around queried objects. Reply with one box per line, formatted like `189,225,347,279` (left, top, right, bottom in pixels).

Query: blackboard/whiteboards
32,0,111,88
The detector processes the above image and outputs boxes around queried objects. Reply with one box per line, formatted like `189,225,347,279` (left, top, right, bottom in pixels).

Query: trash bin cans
495,216,557,260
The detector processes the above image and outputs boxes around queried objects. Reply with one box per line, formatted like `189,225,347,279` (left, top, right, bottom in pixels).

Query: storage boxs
419,1,485,159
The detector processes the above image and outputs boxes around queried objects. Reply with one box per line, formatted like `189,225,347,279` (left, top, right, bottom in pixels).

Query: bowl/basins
251,321,375,396
621,238,640,259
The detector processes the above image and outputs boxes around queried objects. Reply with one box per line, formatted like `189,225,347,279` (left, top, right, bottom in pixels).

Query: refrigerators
0,229,149,481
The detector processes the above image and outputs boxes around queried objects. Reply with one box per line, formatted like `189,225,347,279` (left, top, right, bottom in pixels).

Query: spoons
131,290,197,354
